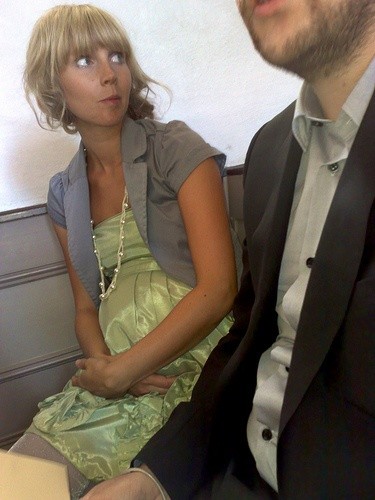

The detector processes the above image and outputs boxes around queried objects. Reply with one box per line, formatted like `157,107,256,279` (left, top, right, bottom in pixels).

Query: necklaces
89,186,131,301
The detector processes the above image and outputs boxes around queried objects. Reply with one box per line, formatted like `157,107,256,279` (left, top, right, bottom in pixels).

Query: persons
84,0,375,500
0,4,237,500
